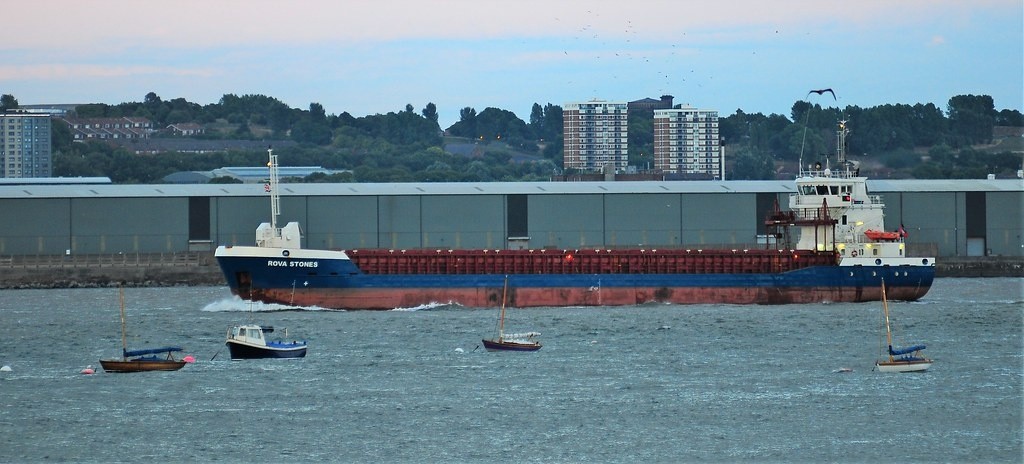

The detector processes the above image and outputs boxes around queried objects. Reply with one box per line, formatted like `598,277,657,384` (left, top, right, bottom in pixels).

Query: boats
225,280,308,358
213,108,937,312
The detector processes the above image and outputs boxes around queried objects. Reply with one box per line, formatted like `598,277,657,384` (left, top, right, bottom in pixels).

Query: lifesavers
851,250,857,257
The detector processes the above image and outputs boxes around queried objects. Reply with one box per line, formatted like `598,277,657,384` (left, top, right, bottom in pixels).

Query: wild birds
806,88,837,101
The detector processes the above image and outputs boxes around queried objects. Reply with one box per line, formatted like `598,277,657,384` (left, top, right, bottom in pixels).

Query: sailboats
98,284,188,373
481,273,542,352
874,277,935,373
